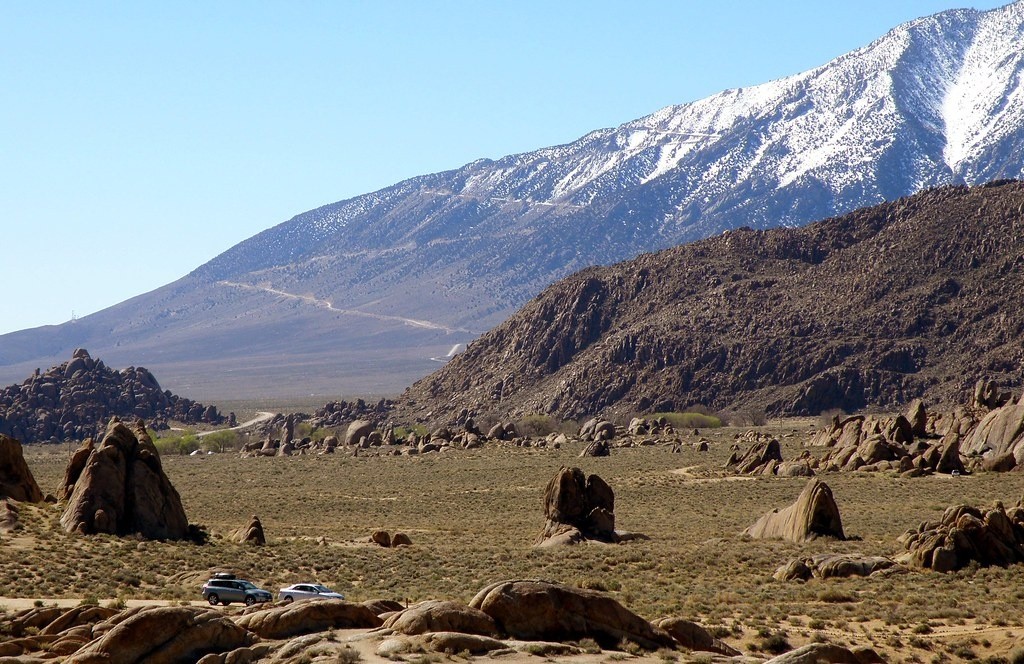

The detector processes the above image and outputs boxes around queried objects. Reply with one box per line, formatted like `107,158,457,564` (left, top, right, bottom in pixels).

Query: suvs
202,572,272,606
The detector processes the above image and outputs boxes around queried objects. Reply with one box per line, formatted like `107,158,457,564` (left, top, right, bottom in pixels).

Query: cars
189,450,203,455
279,584,344,605
208,451,215,455
